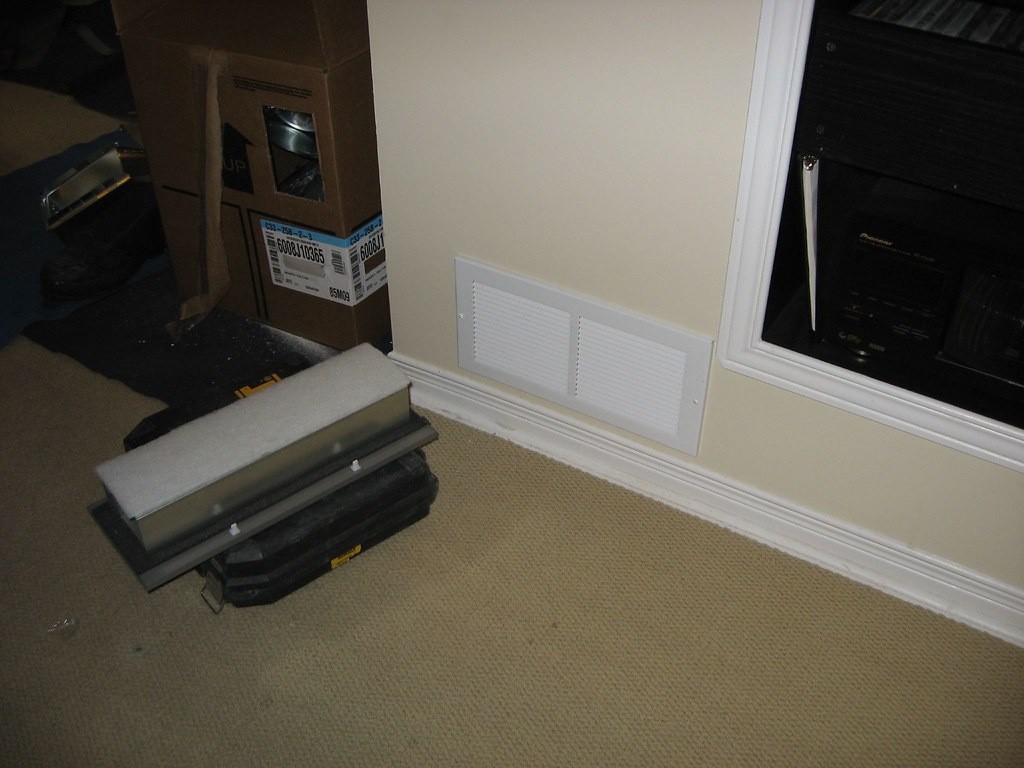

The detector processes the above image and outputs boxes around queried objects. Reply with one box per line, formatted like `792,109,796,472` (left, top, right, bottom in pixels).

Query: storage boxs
111,0,391,352
